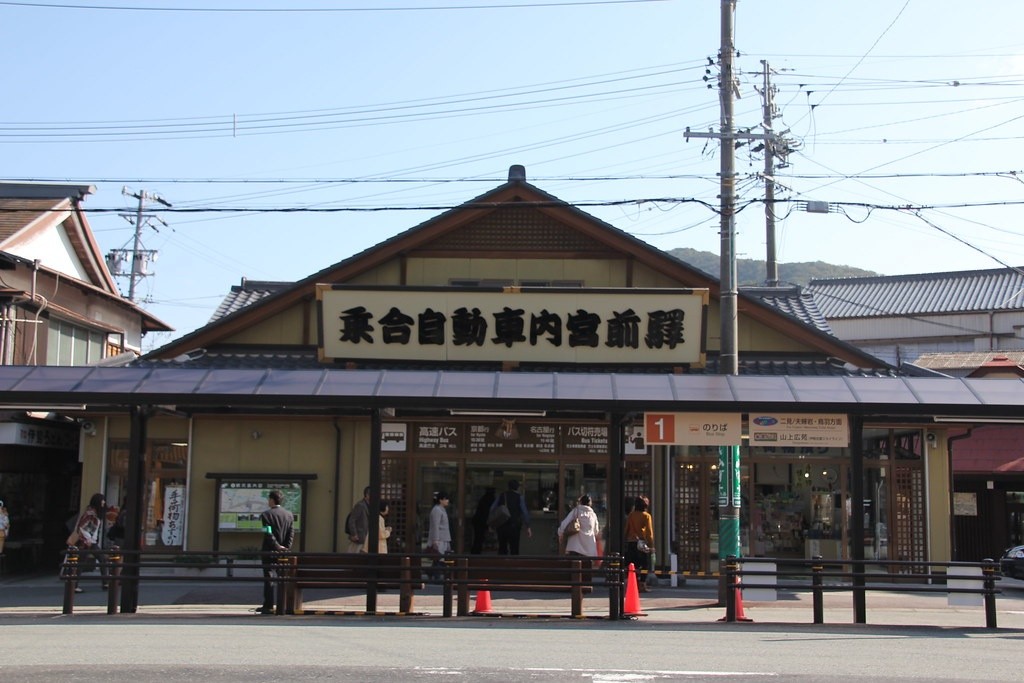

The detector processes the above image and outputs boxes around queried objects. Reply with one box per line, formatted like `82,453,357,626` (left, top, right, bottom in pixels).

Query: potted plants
171,546,278,577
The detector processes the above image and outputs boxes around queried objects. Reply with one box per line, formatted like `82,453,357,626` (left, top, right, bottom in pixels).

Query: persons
115,496,127,585
348,486,392,592
624,496,655,592
256,489,294,612
558,494,599,585
74,493,109,593
427,490,451,578
487,479,531,555
20,504,43,521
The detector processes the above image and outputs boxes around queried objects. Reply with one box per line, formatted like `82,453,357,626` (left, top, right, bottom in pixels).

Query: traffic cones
622,562,649,616
717,578,753,621
474,577,495,611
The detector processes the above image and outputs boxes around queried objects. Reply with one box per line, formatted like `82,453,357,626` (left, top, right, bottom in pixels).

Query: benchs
444,554,592,617
278,556,426,613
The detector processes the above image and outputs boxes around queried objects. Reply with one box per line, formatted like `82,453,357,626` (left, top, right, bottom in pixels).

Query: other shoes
256,601,271,612
103,584,110,590
75,587,86,593
639,582,652,593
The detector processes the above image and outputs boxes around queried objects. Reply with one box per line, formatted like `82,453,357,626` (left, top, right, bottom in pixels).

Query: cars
999,545,1024,581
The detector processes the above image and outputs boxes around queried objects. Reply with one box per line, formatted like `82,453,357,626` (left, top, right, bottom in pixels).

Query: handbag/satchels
564,518,580,537
487,492,511,529
107,523,124,541
637,539,649,552
424,544,441,561
66,529,92,555
